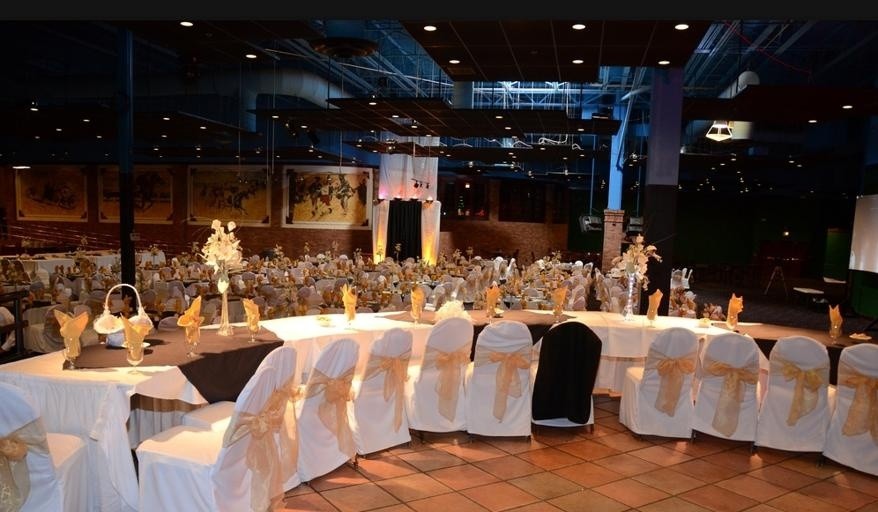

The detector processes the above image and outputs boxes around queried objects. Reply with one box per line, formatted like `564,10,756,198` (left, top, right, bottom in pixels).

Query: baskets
93,284,153,347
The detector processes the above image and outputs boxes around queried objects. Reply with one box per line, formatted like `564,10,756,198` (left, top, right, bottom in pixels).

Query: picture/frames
96,164,175,226
280,163,373,232
185,162,272,228
14,165,89,223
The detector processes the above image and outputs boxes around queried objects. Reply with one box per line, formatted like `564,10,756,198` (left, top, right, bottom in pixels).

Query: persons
308,175,367,217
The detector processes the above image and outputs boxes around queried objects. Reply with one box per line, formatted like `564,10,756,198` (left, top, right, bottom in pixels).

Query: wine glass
552,304,563,324
185,326,202,358
127,341,144,375
344,306,356,330
486,303,496,324
646,308,658,328
247,319,262,344
63,338,81,369
829,325,842,345
726,315,739,333
410,303,422,326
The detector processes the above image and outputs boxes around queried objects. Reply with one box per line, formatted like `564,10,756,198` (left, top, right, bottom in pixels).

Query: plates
850,334,872,340
121,341,151,348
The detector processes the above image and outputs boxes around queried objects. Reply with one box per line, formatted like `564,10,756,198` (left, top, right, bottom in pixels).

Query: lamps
704,119,732,143
